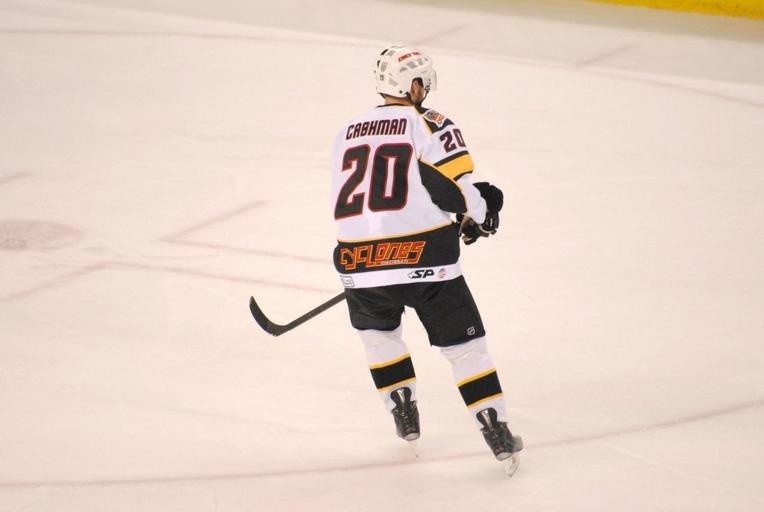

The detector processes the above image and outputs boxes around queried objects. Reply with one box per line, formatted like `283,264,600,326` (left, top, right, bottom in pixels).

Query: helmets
375,45,437,99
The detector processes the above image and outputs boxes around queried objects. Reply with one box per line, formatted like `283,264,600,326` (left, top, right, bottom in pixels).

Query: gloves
456,182,503,244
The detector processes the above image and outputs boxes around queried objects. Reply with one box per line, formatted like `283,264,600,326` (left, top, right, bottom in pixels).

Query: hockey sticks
249,291,346,337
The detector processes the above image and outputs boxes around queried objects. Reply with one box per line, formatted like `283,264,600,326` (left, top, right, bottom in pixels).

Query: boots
476,408,523,459
390,385,421,441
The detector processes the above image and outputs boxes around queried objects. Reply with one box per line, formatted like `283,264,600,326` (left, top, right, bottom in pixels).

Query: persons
326,42,524,462
